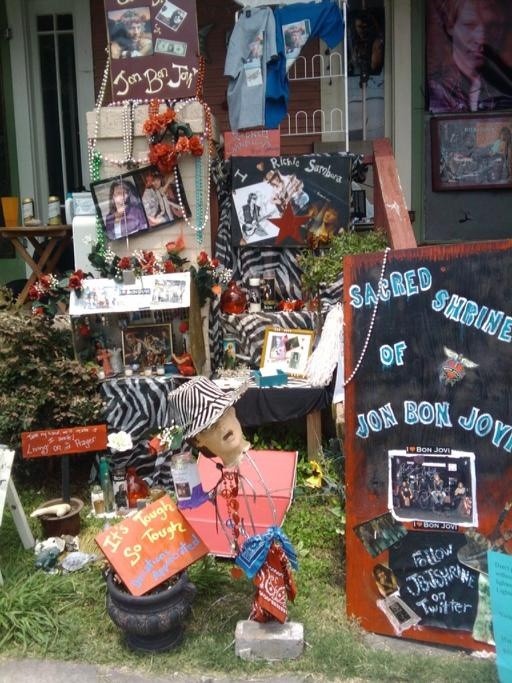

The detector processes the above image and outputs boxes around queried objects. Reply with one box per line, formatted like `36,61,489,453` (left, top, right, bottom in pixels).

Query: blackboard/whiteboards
93,494,211,596
342,239,512,655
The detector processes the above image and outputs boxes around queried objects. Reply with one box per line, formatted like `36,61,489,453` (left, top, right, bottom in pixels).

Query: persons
169,9,185,29
106,174,175,237
400,474,472,516
111,10,152,60
238,169,345,245
125,333,147,358
167,375,250,469
372,564,398,598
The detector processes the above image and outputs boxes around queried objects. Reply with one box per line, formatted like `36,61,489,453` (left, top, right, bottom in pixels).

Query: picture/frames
431,117,512,192
121,324,172,372
91,166,191,244
259,327,315,379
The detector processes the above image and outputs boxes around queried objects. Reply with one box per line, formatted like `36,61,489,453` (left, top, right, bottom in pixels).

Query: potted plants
35,355,106,539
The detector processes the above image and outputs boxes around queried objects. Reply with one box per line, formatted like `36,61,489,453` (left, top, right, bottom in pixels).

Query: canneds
171,451,201,501
48,196,60,226
24,197,33,226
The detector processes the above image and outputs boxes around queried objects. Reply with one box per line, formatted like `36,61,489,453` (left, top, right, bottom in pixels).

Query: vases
107,570,196,651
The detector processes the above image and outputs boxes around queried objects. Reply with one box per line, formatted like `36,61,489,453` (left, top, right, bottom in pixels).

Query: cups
1,197,21,228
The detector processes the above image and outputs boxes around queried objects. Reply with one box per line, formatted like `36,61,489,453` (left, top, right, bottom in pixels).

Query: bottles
65,192,74,225
48,196,62,226
91,452,201,516
221,278,323,313
21,198,35,225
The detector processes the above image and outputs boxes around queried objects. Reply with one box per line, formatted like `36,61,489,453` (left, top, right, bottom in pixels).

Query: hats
164,375,250,442
266,169,278,184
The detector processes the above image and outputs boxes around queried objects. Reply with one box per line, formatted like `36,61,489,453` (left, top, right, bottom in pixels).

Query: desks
0,445,34,580
1,227,70,308
101,375,328,463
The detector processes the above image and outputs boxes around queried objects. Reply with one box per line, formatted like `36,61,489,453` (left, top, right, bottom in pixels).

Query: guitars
242,210,277,237
